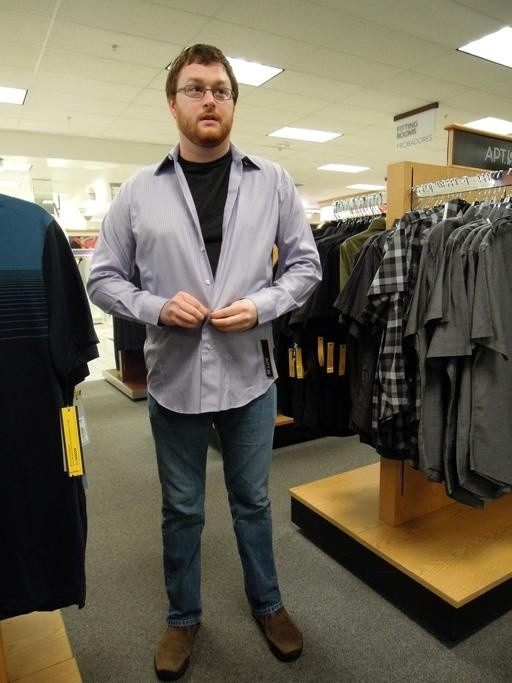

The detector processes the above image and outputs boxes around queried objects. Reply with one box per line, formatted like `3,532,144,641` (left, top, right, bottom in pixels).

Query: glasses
178,85,236,100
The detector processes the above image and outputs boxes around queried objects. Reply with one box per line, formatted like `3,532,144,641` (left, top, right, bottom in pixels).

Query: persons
85,44,324,681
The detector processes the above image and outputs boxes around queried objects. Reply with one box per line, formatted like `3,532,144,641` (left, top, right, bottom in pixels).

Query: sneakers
258,607,302,662
154,621,200,681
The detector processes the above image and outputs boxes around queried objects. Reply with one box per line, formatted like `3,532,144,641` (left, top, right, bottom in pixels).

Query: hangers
332,167,512,227
